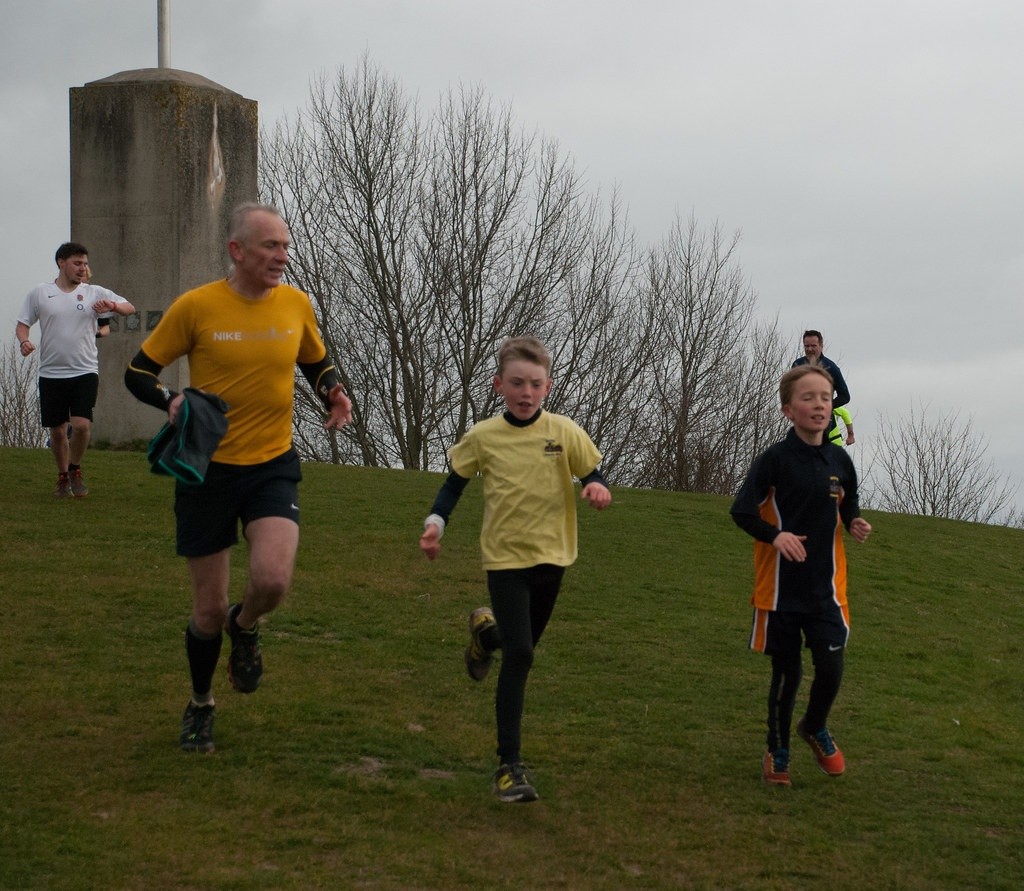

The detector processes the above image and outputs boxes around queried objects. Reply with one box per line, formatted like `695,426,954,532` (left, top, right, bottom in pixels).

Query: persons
791,330,850,410
15,243,136,498
729,364,872,785
828,407,856,449
123,201,352,758
419,335,612,804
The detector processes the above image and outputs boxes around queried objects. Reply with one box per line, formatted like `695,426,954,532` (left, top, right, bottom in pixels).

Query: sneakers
68,469,88,497
465,607,498,681
54,474,74,497
762,749,792,786
180,699,216,754
491,762,539,804
796,719,845,776
222,602,263,694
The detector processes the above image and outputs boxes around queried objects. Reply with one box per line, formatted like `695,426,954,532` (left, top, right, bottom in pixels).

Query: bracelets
20,340,29,347
849,432,854,435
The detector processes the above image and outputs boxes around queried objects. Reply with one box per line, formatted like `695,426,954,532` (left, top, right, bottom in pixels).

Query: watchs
110,301,117,312
